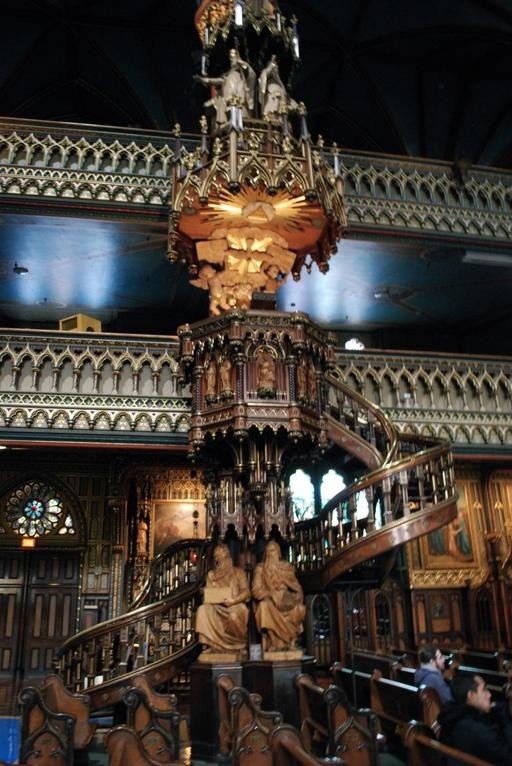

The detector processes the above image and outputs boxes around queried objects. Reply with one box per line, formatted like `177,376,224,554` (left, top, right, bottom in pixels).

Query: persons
414,645,456,703
199,261,234,316
253,541,305,649
438,672,512,764
259,260,289,294
196,542,249,650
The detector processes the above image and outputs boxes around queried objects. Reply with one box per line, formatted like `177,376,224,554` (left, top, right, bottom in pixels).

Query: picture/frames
147,498,207,560
418,477,478,569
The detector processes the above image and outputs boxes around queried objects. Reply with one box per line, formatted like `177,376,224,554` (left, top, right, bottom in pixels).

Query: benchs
213,643,511,766
122,675,191,760
21,674,97,766
107,727,191,766
